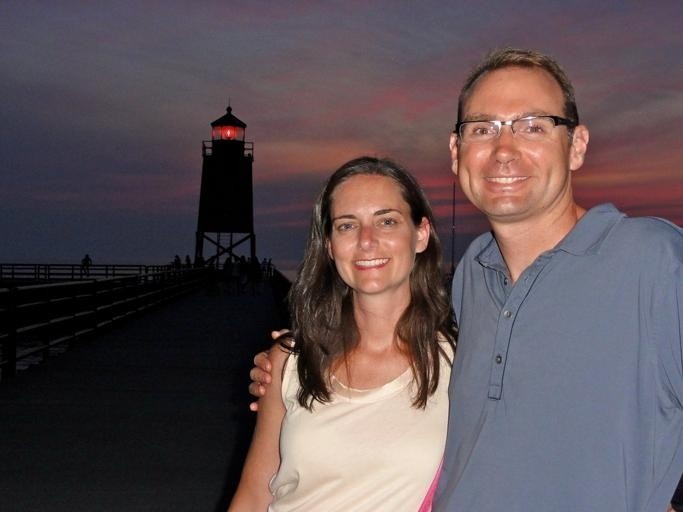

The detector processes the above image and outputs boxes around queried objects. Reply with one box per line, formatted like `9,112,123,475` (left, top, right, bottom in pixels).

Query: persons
228,156,455,511
171,254,275,294
81,254,92,277
250,49,683,511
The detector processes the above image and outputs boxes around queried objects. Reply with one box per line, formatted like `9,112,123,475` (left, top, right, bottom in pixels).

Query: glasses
455,116,574,141
